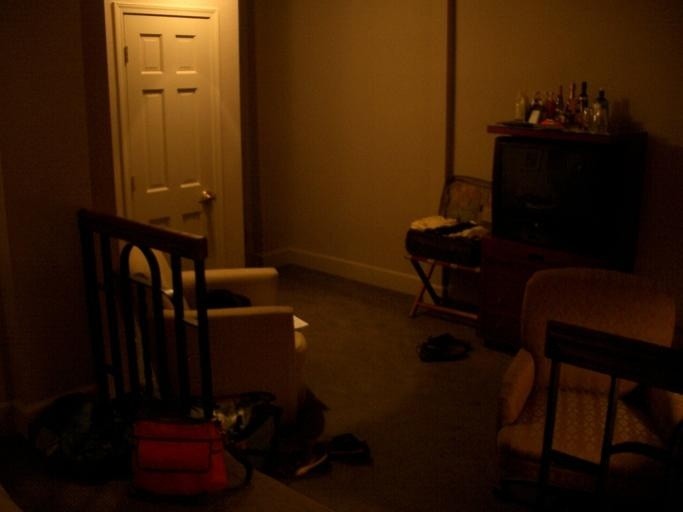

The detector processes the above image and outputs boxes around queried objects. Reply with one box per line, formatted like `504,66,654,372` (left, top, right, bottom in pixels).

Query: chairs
535,320,683,511
76,207,208,416
129,246,309,419
495,268,683,488
403,175,493,319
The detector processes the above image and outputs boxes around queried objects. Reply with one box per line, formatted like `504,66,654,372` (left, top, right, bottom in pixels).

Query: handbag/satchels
131,419,228,495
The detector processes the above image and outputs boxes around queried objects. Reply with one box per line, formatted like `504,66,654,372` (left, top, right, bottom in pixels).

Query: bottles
515,83,609,124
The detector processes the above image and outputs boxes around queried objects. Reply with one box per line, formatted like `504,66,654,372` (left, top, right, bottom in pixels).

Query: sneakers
420,333,469,361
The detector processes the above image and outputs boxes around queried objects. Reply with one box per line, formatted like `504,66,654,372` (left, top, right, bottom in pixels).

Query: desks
1,389,337,512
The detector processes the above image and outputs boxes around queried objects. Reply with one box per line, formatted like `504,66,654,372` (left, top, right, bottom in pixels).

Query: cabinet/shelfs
491,135,640,269
